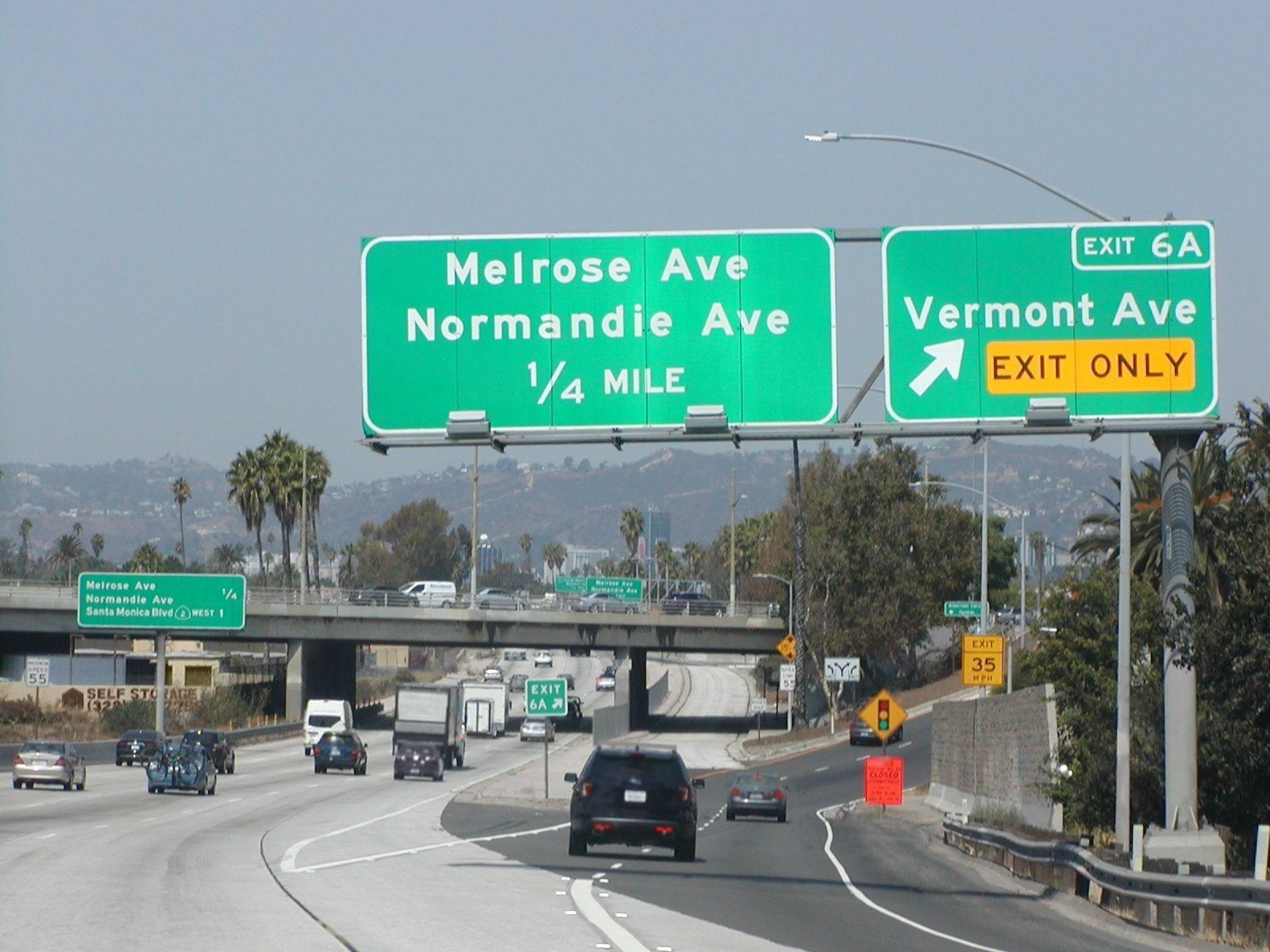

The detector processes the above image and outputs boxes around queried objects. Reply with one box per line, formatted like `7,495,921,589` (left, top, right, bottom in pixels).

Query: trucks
304,698,354,758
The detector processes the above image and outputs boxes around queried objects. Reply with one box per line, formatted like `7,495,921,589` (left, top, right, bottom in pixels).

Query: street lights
912,481,1028,653
301,449,319,602
806,129,1130,852
462,445,480,613
752,572,794,733
730,466,747,618
68,555,86,587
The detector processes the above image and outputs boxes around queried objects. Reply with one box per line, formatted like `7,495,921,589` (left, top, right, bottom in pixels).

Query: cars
14,740,87,792
572,593,728,619
849,706,904,745
995,606,1036,624
725,770,789,822
391,647,616,780
115,728,237,796
349,580,531,614
313,732,368,775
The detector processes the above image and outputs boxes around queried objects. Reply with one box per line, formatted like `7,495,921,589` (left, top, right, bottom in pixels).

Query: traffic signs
825,657,860,683
943,600,990,619
882,216,1219,431
525,679,570,719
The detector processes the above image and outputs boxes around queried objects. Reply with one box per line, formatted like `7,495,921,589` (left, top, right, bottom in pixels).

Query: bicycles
140,738,200,787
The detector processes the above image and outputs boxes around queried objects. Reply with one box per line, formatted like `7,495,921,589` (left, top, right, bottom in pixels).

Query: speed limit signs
27,658,48,687
961,633,1004,685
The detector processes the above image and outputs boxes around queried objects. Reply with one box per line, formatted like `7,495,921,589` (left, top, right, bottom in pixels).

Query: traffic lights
859,689,908,741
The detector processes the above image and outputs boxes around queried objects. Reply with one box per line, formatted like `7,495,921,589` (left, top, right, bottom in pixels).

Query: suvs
564,742,706,866
